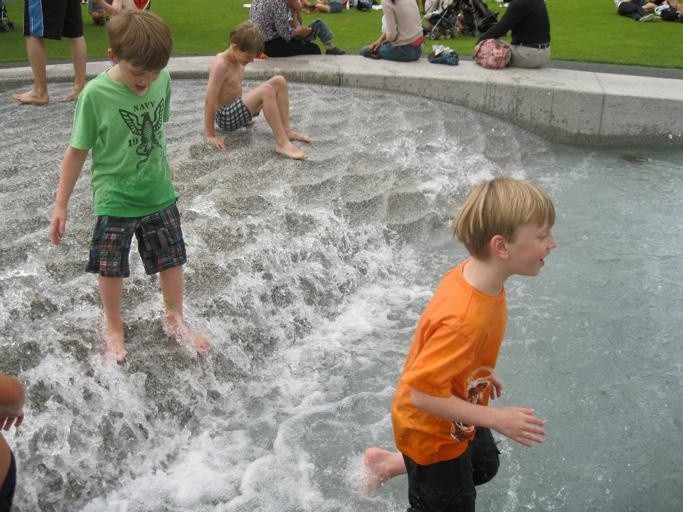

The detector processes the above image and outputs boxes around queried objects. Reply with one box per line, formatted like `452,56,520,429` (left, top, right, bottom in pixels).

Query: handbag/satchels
472,38,512,70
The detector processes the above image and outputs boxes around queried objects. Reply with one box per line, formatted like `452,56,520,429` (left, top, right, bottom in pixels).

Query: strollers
427,0,480,40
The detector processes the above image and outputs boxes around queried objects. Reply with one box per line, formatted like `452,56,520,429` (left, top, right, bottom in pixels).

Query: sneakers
652,16,662,22
326,47,346,55
640,14,654,22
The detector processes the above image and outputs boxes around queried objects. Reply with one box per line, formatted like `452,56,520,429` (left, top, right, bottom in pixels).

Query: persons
306,0,342,14
642,0,666,14
615,0,654,22
363,177,560,512
0,374,25,512
11,0,87,104
96,0,151,15
359,0,424,62
88,0,112,23
287,0,346,53
203,20,310,160
50,8,211,361
474,1,551,69
249,0,321,55
